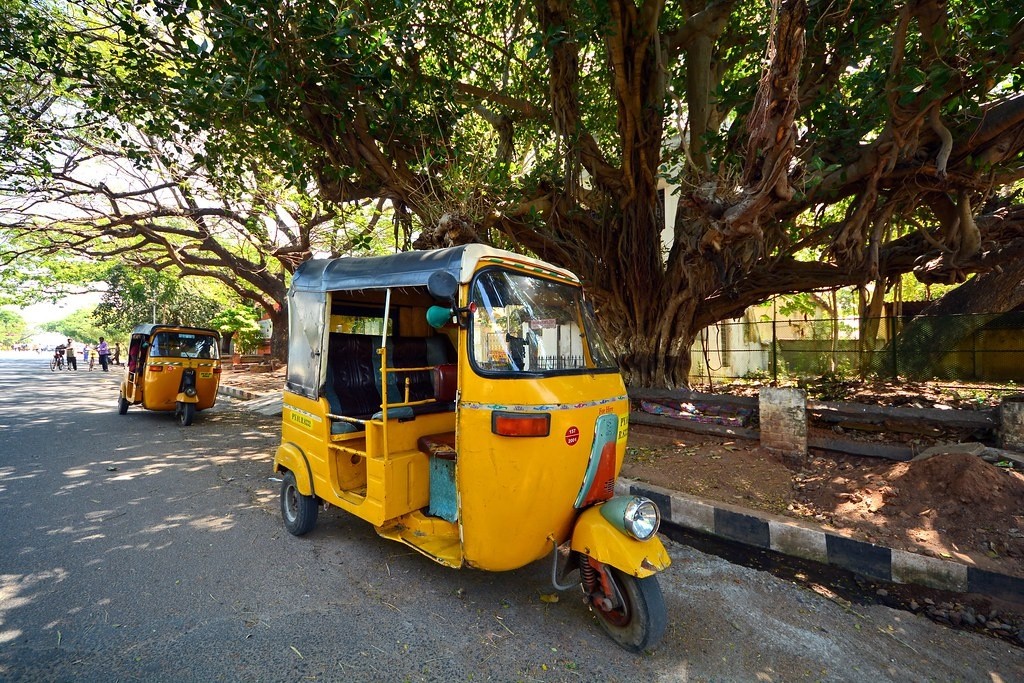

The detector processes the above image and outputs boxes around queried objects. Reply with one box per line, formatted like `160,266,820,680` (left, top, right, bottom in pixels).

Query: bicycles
87,348,98,372
50,348,66,372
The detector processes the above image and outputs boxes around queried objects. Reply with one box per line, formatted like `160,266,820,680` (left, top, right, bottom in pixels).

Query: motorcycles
117,323,223,427
272,243,673,654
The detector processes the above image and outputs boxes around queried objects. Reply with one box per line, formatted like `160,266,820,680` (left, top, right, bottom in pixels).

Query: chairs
416,363,458,523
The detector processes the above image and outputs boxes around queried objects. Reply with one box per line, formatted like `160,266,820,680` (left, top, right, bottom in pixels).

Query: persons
83,344,91,363
95,337,109,372
113,343,120,364
65,338,77,371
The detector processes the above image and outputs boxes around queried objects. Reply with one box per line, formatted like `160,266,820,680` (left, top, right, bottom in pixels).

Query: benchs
323,332,458,490
